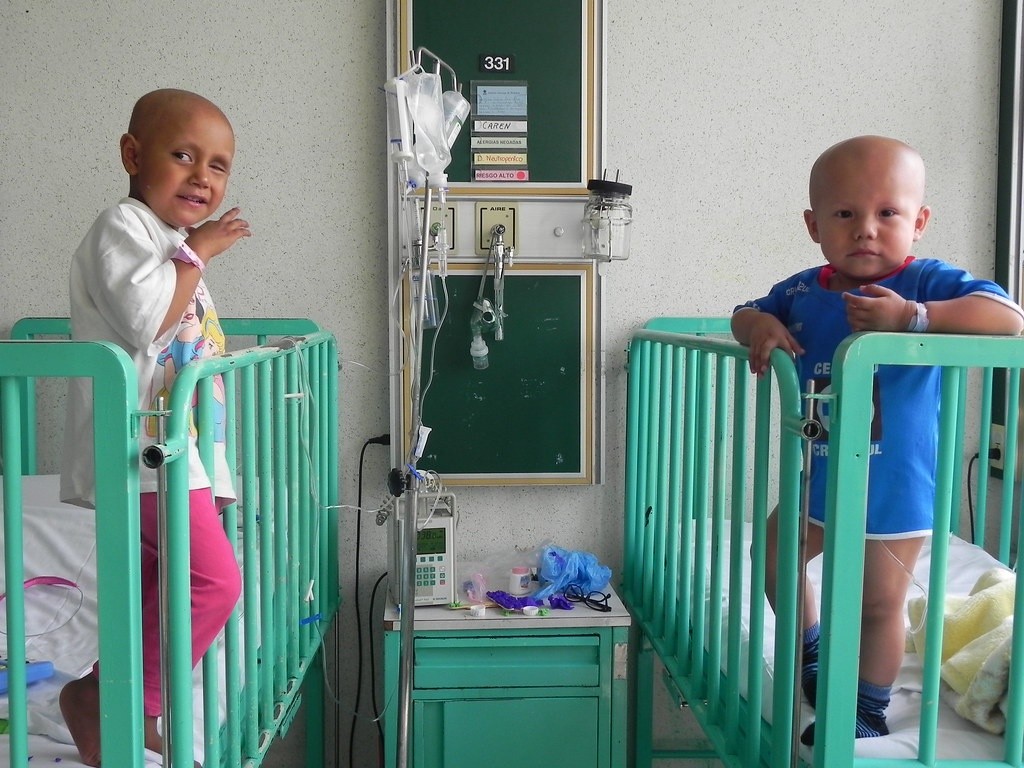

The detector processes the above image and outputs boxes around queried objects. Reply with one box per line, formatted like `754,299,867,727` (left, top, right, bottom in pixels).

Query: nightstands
384,567,630,768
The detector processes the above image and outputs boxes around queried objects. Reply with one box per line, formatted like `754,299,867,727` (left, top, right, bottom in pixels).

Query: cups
508,567,531,594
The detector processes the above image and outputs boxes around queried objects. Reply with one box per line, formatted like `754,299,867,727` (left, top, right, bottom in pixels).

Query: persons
730,135,1023,747
58,88,251,768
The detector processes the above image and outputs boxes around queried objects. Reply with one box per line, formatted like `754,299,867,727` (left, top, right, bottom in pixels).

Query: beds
621,311,1023,768
0,317,346,767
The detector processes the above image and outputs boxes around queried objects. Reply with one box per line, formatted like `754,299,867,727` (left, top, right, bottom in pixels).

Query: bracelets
907,302,929,333
169,238,207,274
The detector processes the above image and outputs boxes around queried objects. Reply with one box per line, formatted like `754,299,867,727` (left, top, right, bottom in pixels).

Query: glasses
563,584,612,612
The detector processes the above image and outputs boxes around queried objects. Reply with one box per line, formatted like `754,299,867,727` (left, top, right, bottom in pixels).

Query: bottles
587,180,632,262
433,91,470,150
